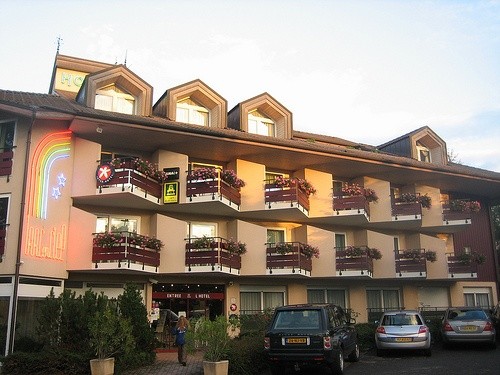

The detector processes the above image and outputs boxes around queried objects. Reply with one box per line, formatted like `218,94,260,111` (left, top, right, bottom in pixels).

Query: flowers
344,182,379,204
186,167,245,191
449,200,481,214
275,176,317,196
344,244,383,262
401,247,437,264
274,242,320,260
93,229,166,254
192,236,248,257
399,191,433,210
96,157,169,181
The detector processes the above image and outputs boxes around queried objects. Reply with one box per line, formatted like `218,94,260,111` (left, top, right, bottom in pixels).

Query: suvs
264,303,360,375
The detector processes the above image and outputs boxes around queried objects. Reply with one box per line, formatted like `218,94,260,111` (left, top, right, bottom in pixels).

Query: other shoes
178,361,186,366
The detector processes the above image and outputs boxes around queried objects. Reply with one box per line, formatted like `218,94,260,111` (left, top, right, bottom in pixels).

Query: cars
374,311,431,357
441,307,496,349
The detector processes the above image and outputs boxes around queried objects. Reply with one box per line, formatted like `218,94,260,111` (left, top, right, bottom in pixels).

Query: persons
172,315,191,366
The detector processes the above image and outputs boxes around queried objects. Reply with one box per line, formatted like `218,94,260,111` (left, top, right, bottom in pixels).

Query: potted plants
87,310,136,375
182,314,241,375
